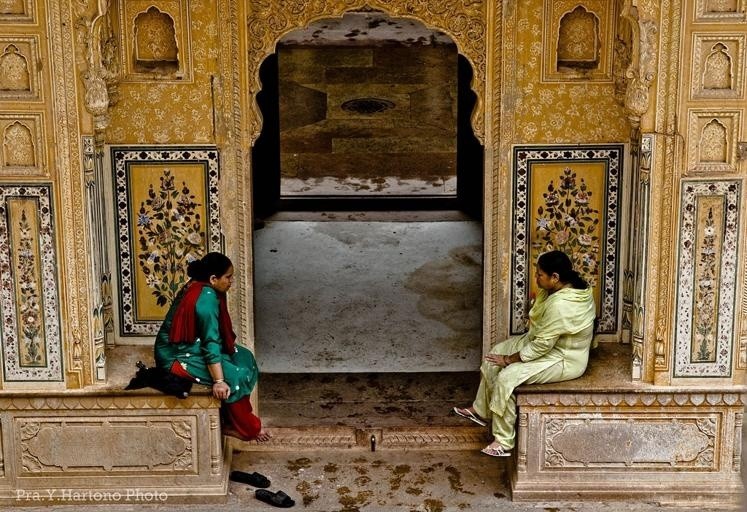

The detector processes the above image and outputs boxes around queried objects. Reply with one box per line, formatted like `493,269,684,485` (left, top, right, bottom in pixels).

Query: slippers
480,443,513,457
255,489,296,508
452,405,488,428
229,470,271,488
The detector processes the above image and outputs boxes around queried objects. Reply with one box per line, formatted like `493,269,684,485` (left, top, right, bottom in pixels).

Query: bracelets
213,379,224,384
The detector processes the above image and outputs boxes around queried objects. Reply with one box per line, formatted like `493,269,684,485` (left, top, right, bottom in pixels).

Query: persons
155,252,272,442
454,251,596,457
